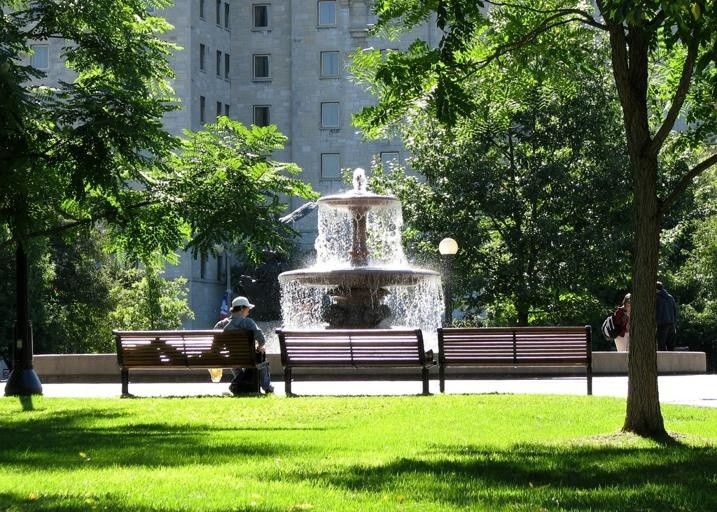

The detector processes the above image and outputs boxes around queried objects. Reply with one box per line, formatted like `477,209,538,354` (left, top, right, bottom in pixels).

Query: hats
232,296,255,309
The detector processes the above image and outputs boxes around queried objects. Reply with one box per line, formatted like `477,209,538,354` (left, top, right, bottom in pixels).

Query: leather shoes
265,386,274,393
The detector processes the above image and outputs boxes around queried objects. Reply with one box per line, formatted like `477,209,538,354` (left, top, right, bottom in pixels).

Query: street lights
438,237,458,327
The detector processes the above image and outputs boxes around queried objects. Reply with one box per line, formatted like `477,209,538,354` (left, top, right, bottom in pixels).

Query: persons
653,281,678,351
611,293,629,352
209,295,274,396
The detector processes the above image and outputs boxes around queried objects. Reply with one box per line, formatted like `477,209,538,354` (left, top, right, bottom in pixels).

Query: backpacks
601,308,627,341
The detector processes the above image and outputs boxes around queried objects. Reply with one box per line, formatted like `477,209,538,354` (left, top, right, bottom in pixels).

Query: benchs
112,328,271,398
437,325,593,394
274,327,437,396
